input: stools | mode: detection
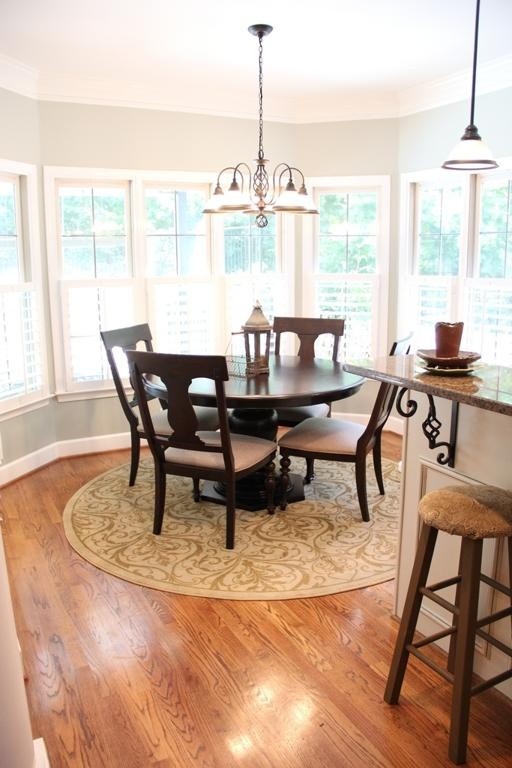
[384,484,512,765]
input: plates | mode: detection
[416,349,482,365]
[418,360,475,377]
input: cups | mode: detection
[434,321,464,358]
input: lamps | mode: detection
[201,23,320,229]
[440,0,500,172]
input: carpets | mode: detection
[60,447,402,601]
